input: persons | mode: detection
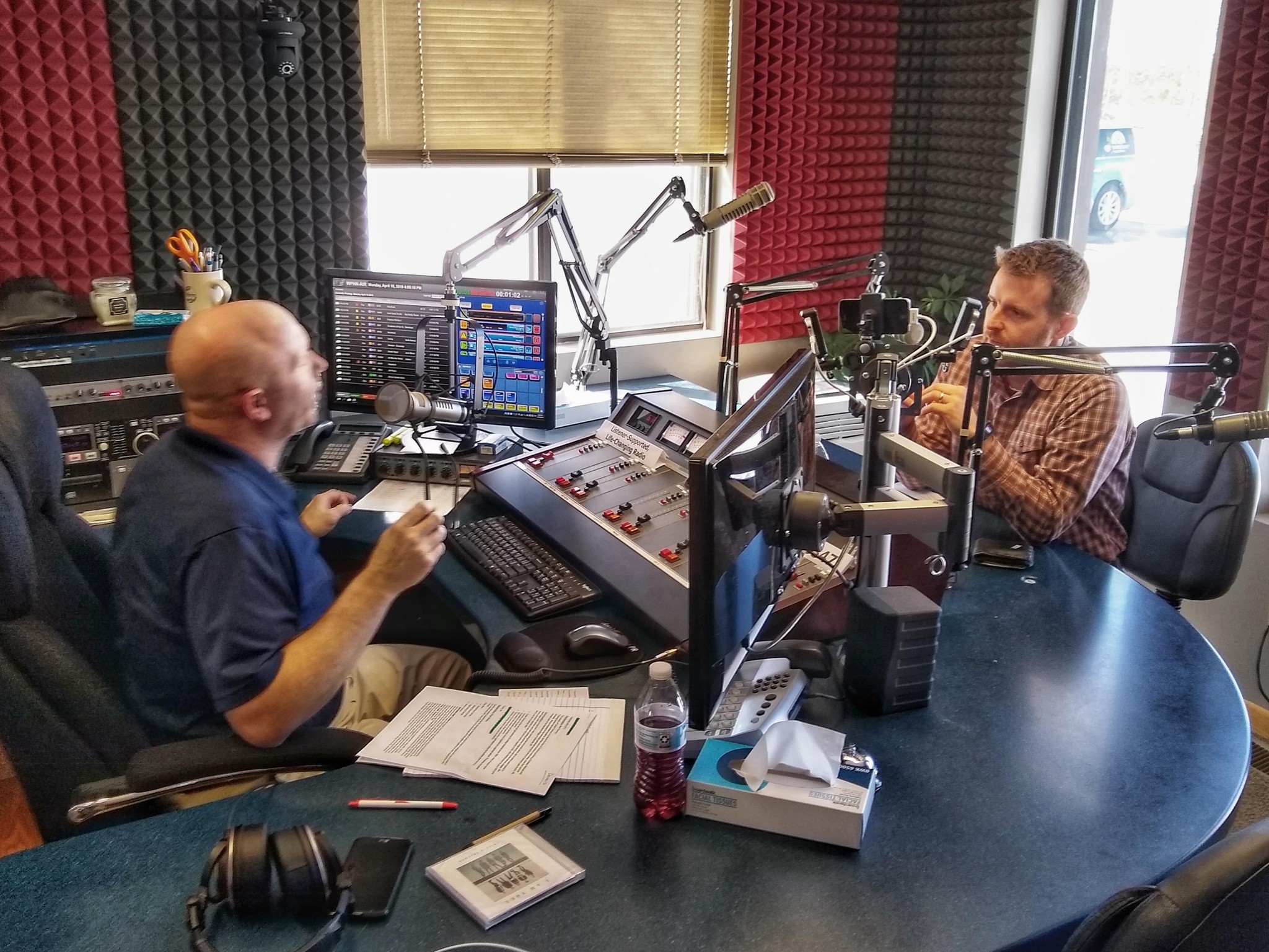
[472,849,535,894]
[895,239,1137,574]
[110,300,471,811]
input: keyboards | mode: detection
[447,512,602,624]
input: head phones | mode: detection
[183,823,355,950]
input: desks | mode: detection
[0,377,1251,952]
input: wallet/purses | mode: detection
[971,538,1035,570]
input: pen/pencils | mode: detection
[463,807,556,852]
[347,799,460,808]
[178,239,224,272]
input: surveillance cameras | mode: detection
[259,20,305,79]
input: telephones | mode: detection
[644,637,833,755]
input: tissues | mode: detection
[685,720,879,852]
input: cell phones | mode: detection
[336,835,411,920]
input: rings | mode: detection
[939,393,945,404]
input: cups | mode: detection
[182,268,232,315]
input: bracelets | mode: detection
[964,423,994,446]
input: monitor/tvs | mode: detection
[687,341,831,732]
[322,268,557,430]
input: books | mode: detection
[425,822,586,930]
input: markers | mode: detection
[382,423,411,446]
[167,227,201,266]
[394,428,413,445]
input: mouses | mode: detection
[562,622,630,659]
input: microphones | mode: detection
[374,381,470,425]
[701,183,775,231]
[1152,410,1269,442]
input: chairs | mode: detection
[1110,415,1261,613]
[1061,821,1269,952]
[0,362,372,850]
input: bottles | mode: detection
[89,276,136,326]
[635,661,689,820]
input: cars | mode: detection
[1086,128,1139,232]
